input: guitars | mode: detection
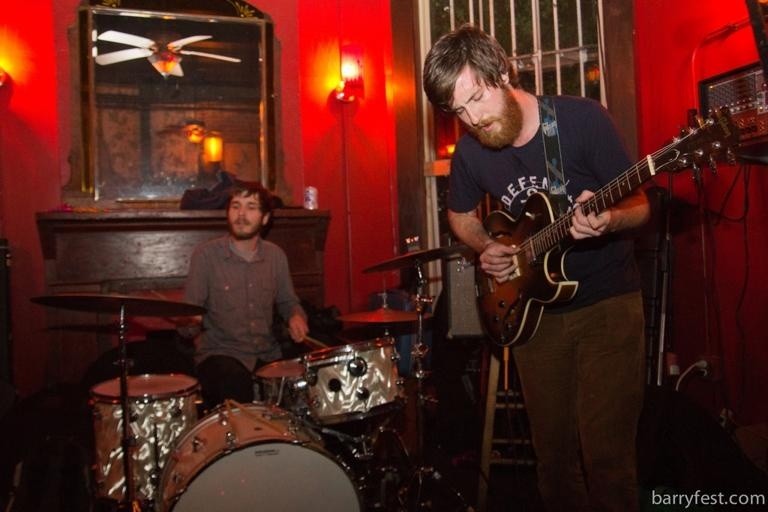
[473,107,741,347]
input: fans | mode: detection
[95,21,242,80]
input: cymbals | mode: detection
[30,291,208,317]
[336,307,432,323]
[362,245,470,272]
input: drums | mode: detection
[152,402,368,512]
[253,358,306,418]
[304,336,407,426]
[90,374,204,502]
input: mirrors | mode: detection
[80,6,272,210]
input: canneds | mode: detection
[303,185,319,210]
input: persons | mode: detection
[173,180,309,413]
[422,21,653,511]
